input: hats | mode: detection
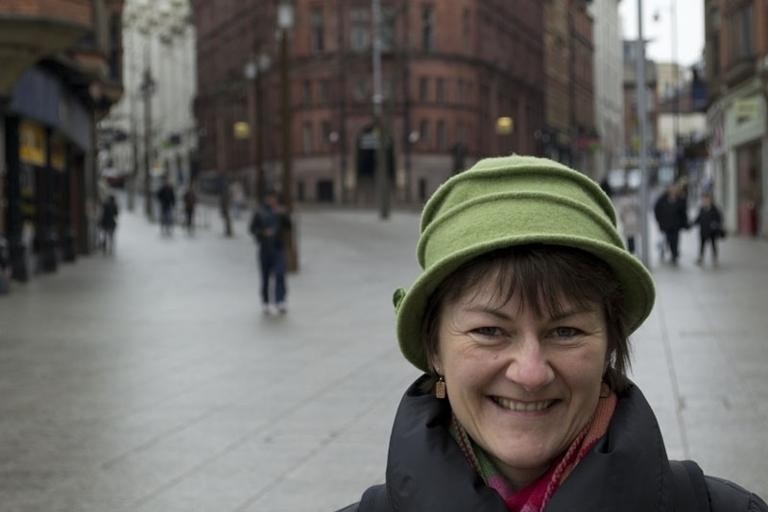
[395,154,655,375]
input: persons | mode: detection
[336,151,767,511]
[615,170,727,269]
[249,188,293,316]
[154,172,245,239]
[99,195,118,253]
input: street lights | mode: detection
[137,67,158,213]
[276,5,296,205]
[242,55,272,199]
